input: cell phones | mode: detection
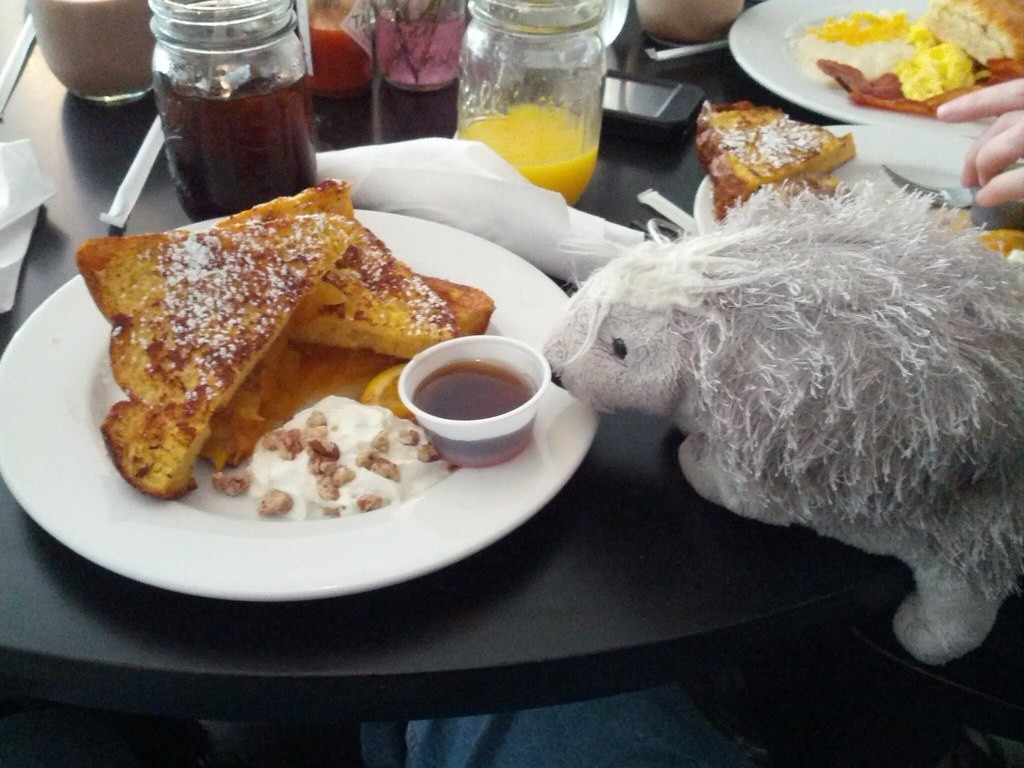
[552,66,706,149]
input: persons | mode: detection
[937,77,1024,206]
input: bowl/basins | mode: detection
[397,330,554,467]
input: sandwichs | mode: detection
[79,178,493,501]
[698,98,859,220]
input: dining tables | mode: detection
[0,1,1022,768]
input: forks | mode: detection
[879,162,981,211]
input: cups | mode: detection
[288,0,373,98]
[145,1,318,223]
[26,0,157,102]
[454,1,605,208]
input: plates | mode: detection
[694,122,1024,278]
[724,0,1024,127]
[2,207,602,602]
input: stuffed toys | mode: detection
[543,177,1024,665]
